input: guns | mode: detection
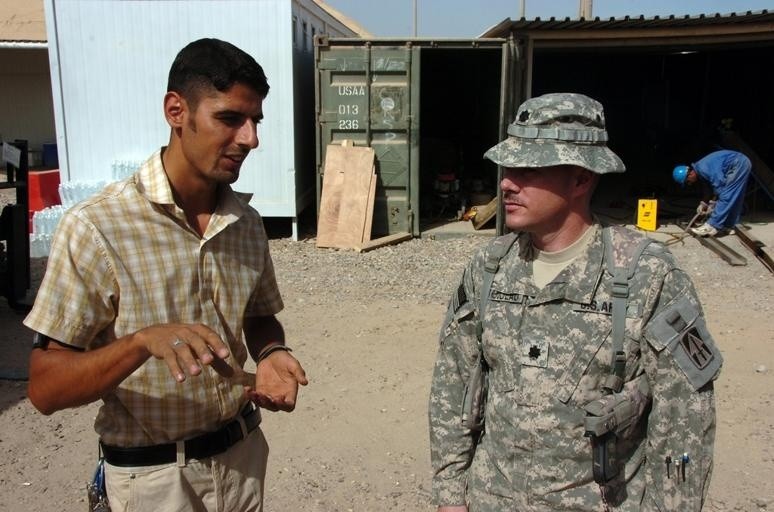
[584,393,626,483]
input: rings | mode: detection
[174,338,184,346]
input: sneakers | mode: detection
[690,223,722,237]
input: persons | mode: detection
[673,148,752,237]
[22,36,308,511]
[427,90,723,511]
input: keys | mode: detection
[83,479,111,511]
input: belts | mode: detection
[99,397,262,467]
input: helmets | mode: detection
[672,165,690,189]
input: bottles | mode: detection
[29,159,148,259]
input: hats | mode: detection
[481,91,628,174]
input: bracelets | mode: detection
[256,344,293,364]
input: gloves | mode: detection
[696,199,717,216]
